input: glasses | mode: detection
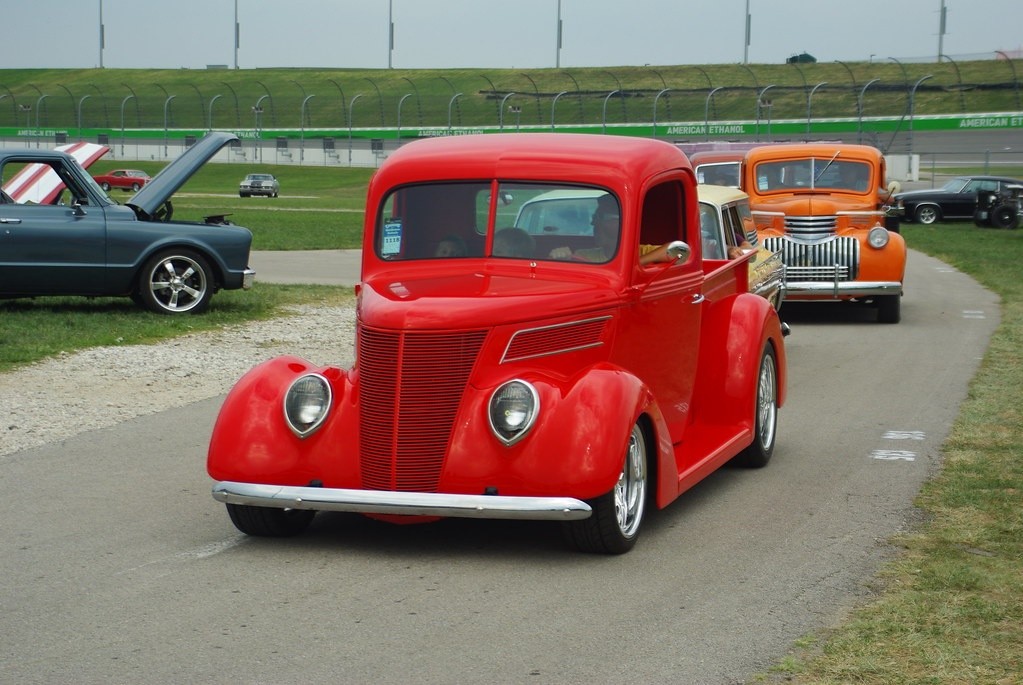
[591,211,619,226]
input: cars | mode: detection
[728,141,907,325]
[690,148,795,197]
[92,169,152,193]
[785,54,816,64]
[889,174,1023,226]
[205,133,791,559]
[0,132,256,317]
[513,179,789,314]
[239,173,280,198]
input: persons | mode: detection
[703,237,744,260]
[996,184,1012,198]
[703,209,756,264]
[435,234,466,257]
[550,193,676,265]
[714,175,727,185]
[833,162,894,205]
[493,228,536,258]
[121,171,129,178]
